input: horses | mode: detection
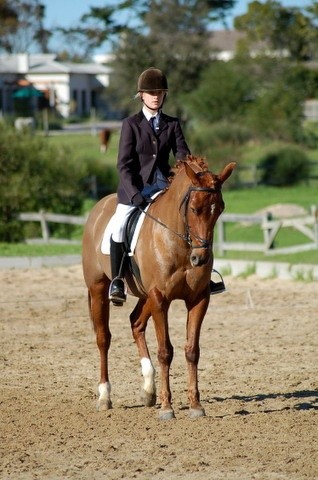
[97,129,110,152]
[82,154,236,419]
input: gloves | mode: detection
[132,193,146,206]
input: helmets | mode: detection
[137,67,169,91]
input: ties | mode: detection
[149,117,156,136]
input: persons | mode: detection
[109,67,225,307]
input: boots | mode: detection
[110,233,126,306]
[210,279,225,294]
